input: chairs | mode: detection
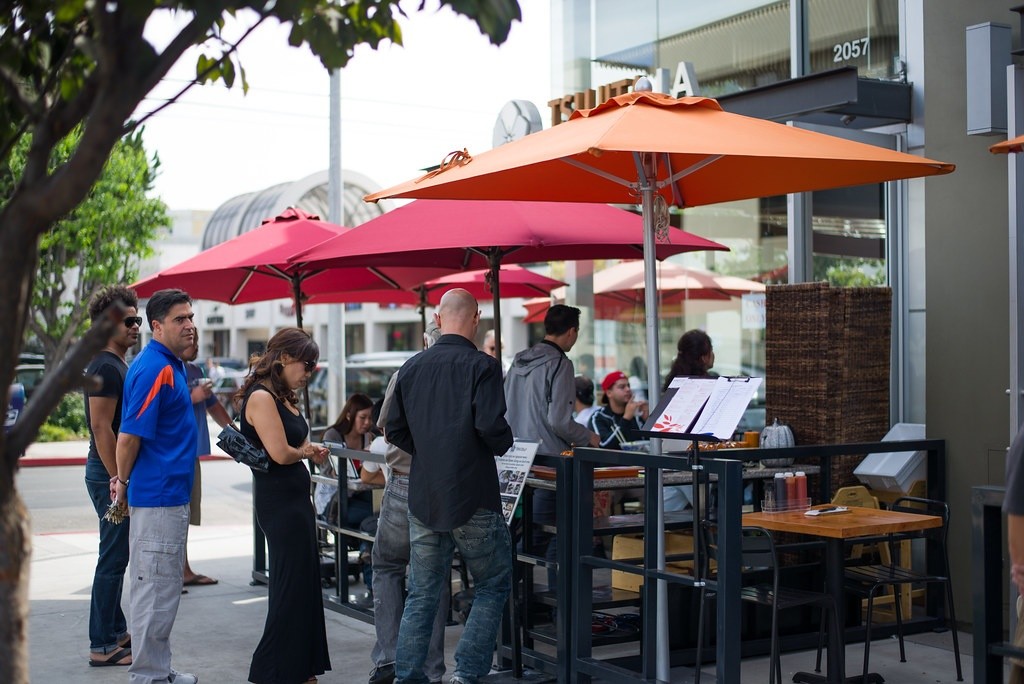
[696,490,963,684]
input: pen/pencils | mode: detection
[818,507,837,512]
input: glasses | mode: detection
[303,361,316,374]
[112,316,143,328]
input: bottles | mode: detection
[775,471,807,512]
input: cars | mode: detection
[191,357,246,421]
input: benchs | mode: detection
[318,502,721,650]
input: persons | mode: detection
[109,289,241,684]
[84,284,143,668]
[313,325,506,684]
[233,326,331,684]
[503,305,752,513]
[383,287,514,684]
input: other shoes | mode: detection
[156,670,198,684]
[369,665,395,684]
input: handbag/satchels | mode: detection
[216,424,273,474]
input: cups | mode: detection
[199,378,211,399]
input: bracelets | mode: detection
[118,478,129,485]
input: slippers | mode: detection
[89,648,135,666]
[117,637,133,648]
[182,573,219,584]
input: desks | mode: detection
[311,474,385,596]
[740,504,942,684]
[522,465,821,649]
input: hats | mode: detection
[601,372,627,404]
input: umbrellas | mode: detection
[126,198,770,453]
[362,78,954,682]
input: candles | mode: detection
[744,429,759,447]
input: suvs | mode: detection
[299,351,419,423]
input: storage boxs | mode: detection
[853,423,927,493]
